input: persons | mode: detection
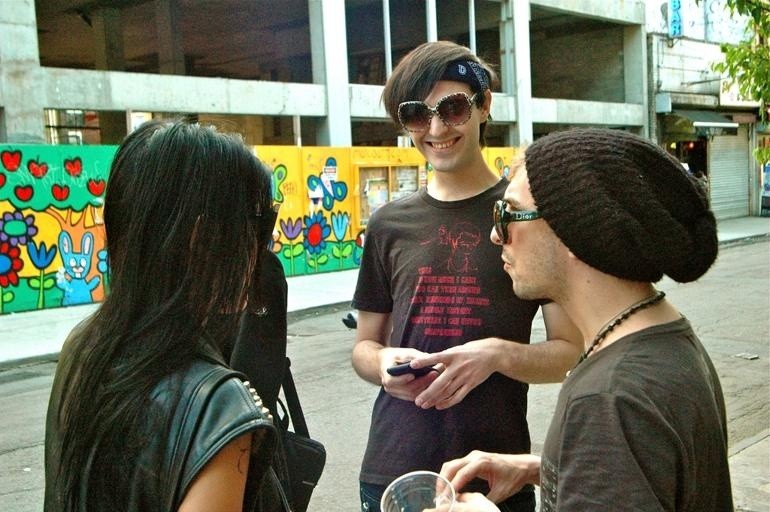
[44,117,277,512]
[423,127,732,511]
[348,41,586,511]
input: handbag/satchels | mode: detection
[270,429,326,511]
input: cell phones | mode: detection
[387,362,429,377]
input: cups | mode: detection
[379,469,456,512]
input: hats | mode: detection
[525,128,718,282]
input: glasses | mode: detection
[493,199,542,244]
[397,92,478,133]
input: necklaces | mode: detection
[572,289,665,368]
